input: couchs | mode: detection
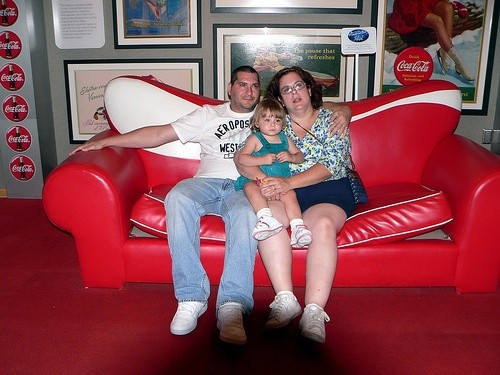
[42,75,500,294]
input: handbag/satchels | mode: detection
[346,170,368,204]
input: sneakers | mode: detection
[300,303,330,344]
[170,301,208,335]
[217,302,247,343]
[266,292,302,328]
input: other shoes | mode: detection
[252,215,283,241]
[290,225,312,248]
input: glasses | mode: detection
[279,81,306,96]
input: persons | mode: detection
[234,66,356,342]
[69,65,353,345]
[238,98,312,248]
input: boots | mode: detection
[436,47,450,72]
[448,46,475,81]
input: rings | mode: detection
[342,125,346,128]
[272,185,276,190]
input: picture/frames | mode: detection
[213,23,359,102]
[63,58,203,144]
[112,0,202,49]
[367,0,500,116]
[210,0,363,15]
